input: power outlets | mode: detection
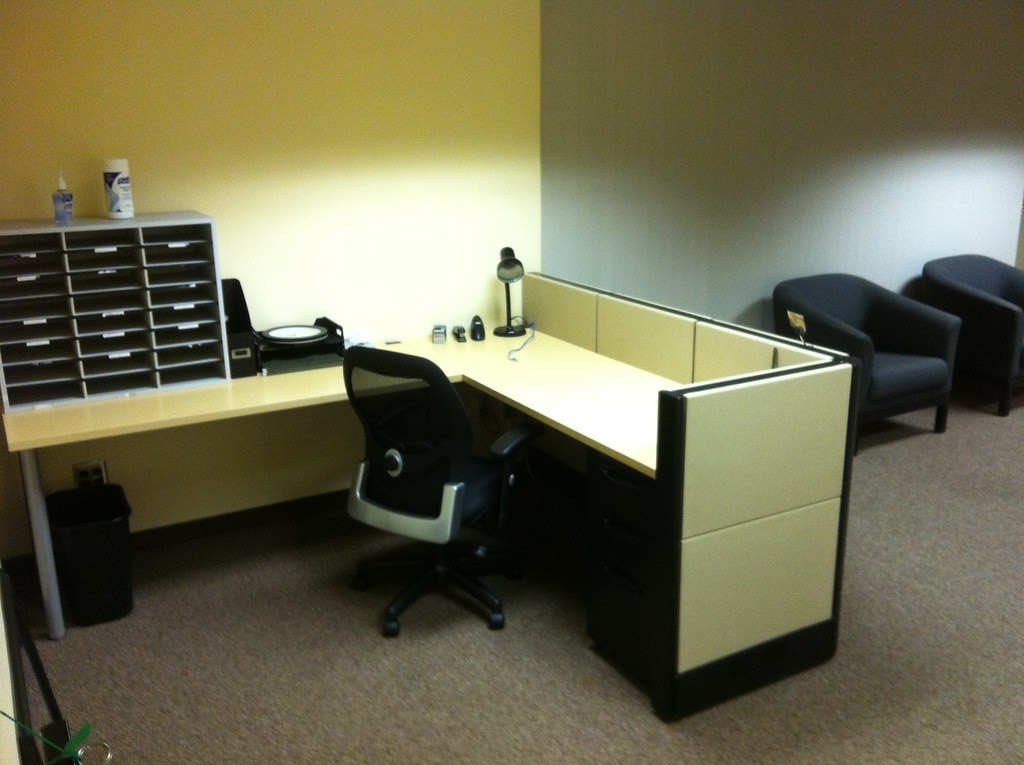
[72,459,107,488]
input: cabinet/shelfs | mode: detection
[1,209,232,412]
[586,447,657,695]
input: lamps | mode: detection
[494,246,526,337]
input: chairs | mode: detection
[923,254,1024,416]
[773,272,962,455]
[343,345,536,636]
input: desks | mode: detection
[0,270,861,723]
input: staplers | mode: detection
[452,325,467,343]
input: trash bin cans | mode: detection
[44,483,134,627]
